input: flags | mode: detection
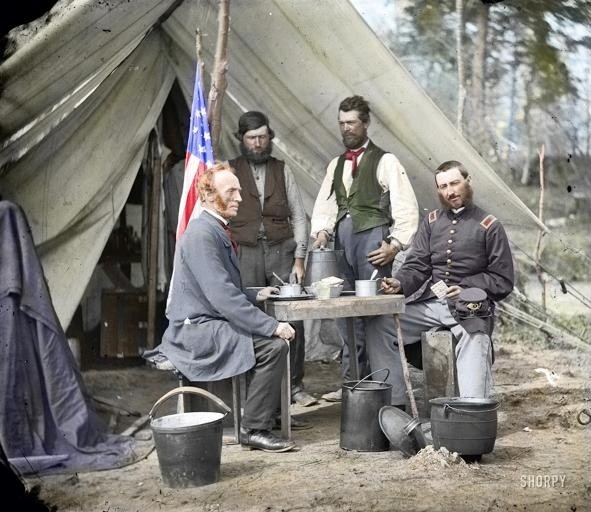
[177,66,215,246]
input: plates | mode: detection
[267,294,315,300]
[341,291,356,295]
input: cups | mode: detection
[356,278,386,297]
[318,282,331,300]
[274,284,302,296]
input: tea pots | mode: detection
[305,246,354,295]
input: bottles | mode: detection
[111,225,144,251]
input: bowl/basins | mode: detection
[303,286,344,298]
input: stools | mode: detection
[173,370,254,448]
[417,326,464,404]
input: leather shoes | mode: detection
[289,383,317,407]
[273,414,313,431]
[321,391,342,402]
[240,428,296,453]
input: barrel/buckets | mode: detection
[302,247,340,287]
[339,379,392,452]
[149,411,226,489]
[429,395,495,454]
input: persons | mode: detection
[158,163,295,451]
[365,161,514,412]
[225,111,317,406]
[310,95,419,411]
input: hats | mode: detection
[455,287,493,320]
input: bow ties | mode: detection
[342,152,357,180]
[226,226,239,256]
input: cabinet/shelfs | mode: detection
[97,288,168,365]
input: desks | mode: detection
[256,287,427,443]
